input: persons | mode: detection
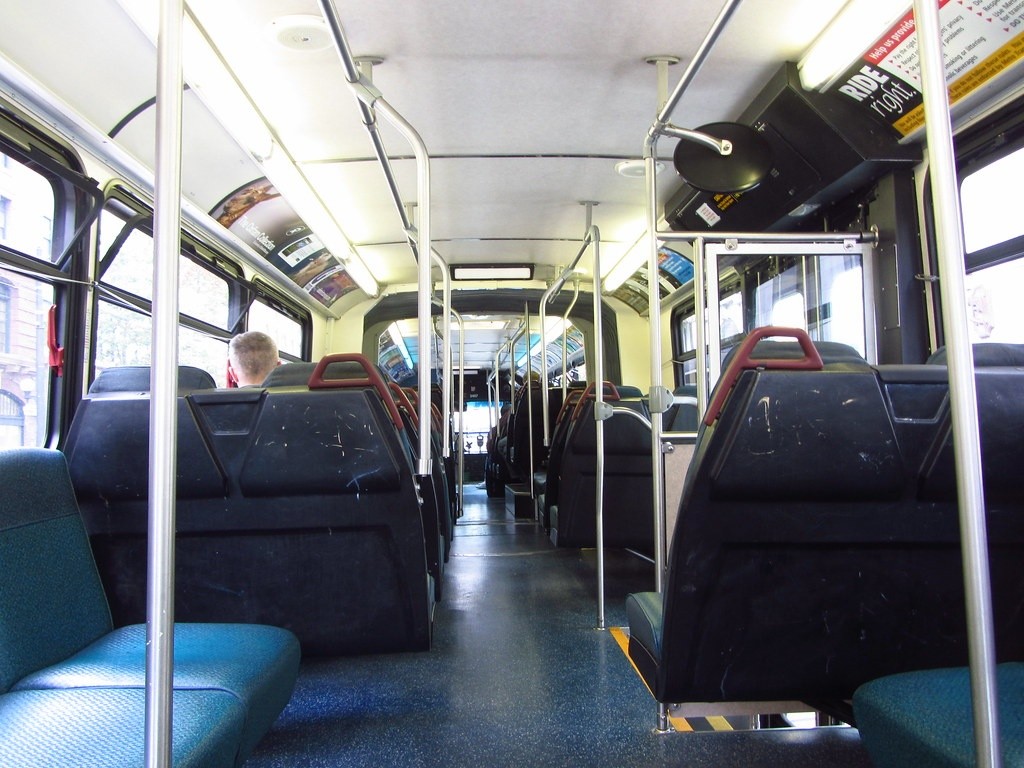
[228,330,282,390]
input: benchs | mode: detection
[626,325,1022,699]
[65,353,458,653]
[852,660,1024,768]
[0,449,300,768]
[485,381,698,546]
